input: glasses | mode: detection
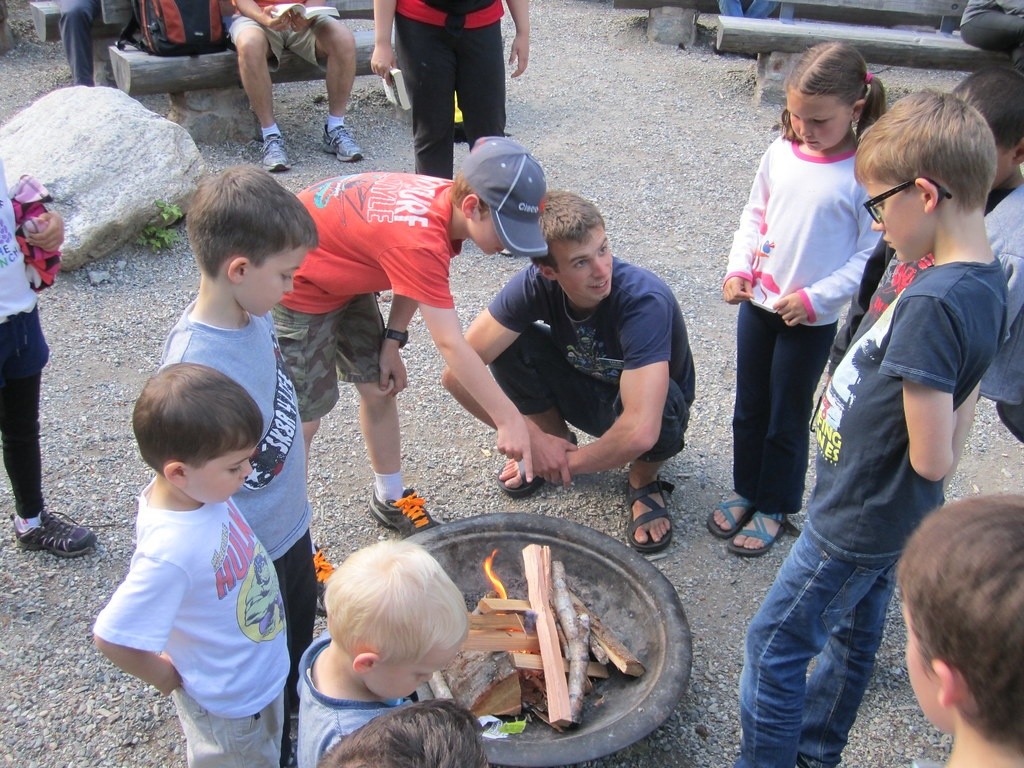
[862,176,953,224]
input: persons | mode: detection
[898,496,1024,768]
[159,165,318,768]
[58,0,95,86]
[0,157,96,558]
[92,364,290,768]
[961,1,1024,73]
[371,0,529,256]
[319,700,489,768]
[297,541,469,768]
[224,0,364,171]
[442,189,695,552]
[707,41,885,557]
[273,140,550,619]
[734,68,1024,768]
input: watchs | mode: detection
[384,328,408,348]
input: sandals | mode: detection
[495,431,578,497]
[626,472,677,551]
[726,509,787,555]
[706,491,755,539]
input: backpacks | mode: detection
[117,0,232,58]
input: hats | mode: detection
[462,136,549,257]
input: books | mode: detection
[382,67,410,110]
[270,3,340,20]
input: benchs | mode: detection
[614,0,1011,108]
[30,0,402,145]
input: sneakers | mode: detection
[259,129,291,172]
[367,486,442,539]
[321,123,363,162]
[10,504,98,558]
[311,544,336,616]
